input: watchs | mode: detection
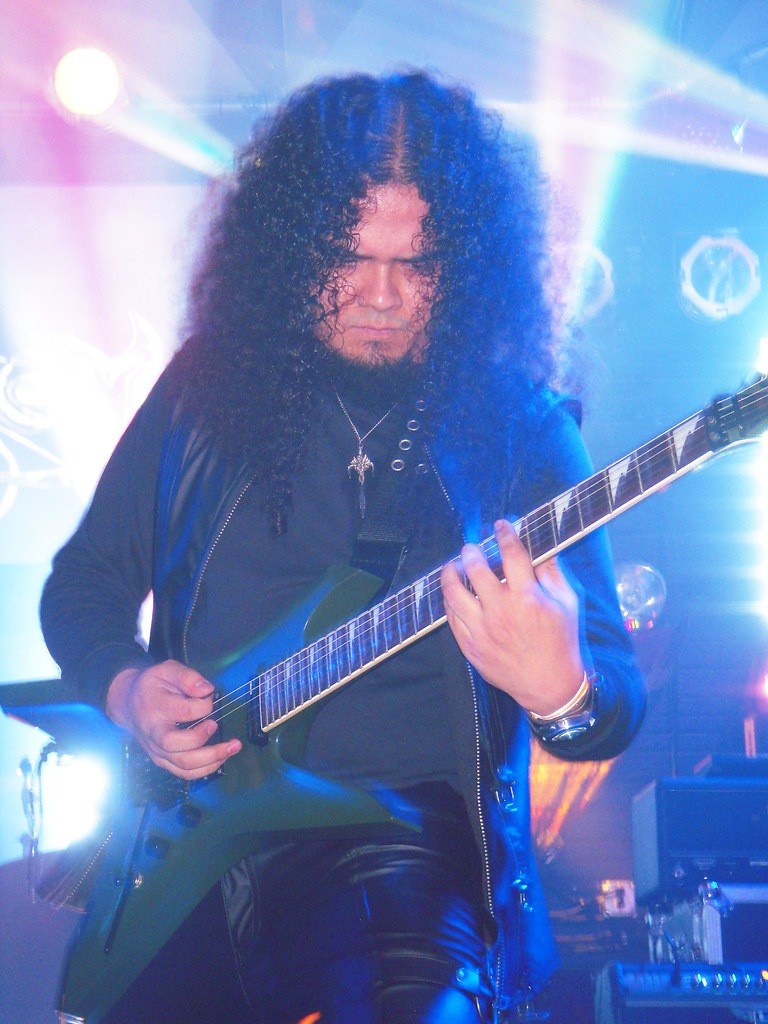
[521,673,599,744]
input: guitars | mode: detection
[2,376,766,1023]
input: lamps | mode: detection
[55,43,122,118]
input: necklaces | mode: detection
[323,371,407,487]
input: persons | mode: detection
[40,65,648,1024]
